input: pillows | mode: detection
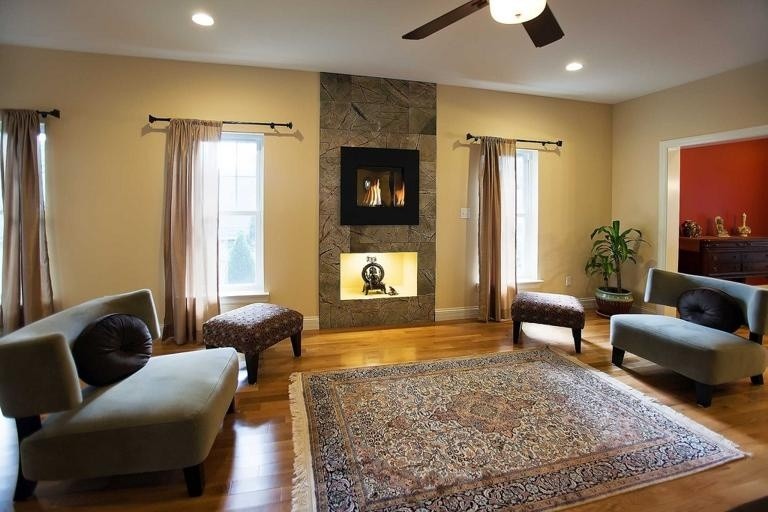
[677,287,744,334]
[72,313,153,388]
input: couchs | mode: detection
[609,268,768,409]
[0,288,239,502]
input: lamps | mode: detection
[487,0,547,25]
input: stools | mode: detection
[511,291,585,354]
[202,302,304,384]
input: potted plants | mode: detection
[584,220,651,319]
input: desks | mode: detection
[679,235,768,279]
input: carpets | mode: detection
[287,342,751,512]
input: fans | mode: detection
[401,0,565,48]
